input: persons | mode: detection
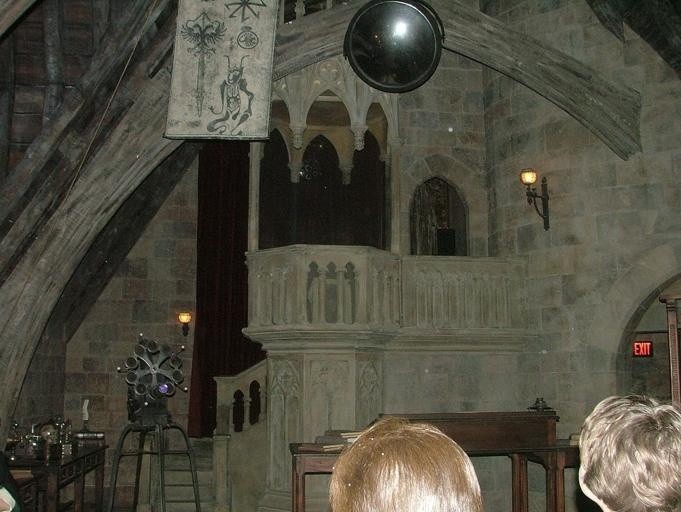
[0,450,24,512]
[574,391,681,512]
[328,415,485,512]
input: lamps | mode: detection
[518,169,550,231]
[178,311,191,337]
